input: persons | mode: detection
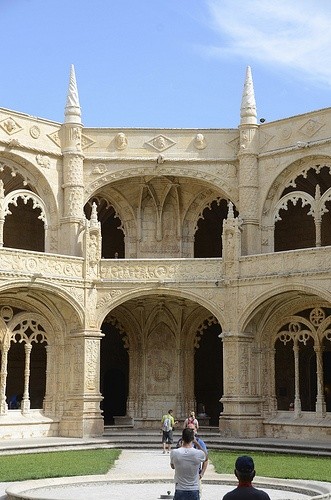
[183,411,198,430]
[170,428,208,500]
[222,455,270,500]
[160,409,178,454]
[176,422,208,499]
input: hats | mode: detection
[235,455,256,475]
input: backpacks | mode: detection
[186,419,196,434]
[162,415,173,432]
